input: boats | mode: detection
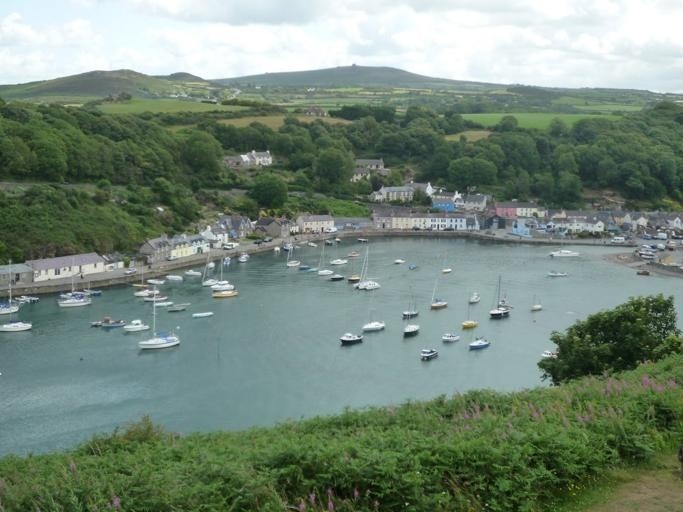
[418,345,438,361]
[272,246,280,252]
[191,312,213,319]
[408,263,418,270]
[237,250,249,262]
[146,277,165,285]
[541,351,558,359]
[546,270,567,277]
[440,267,451,274]
[468,335,490,349]
[0,322,33,332]
[529,304,542,311]
[87,314,150,331]
[165,274,183,281]
[15,295,39,304]
[182,269,201,277]
[143,295,185,312]
[461,319,479,328]
[360,320,384,331]
[439,333,459,342]
[338,332,363,342]
[469,289,480,304]
[393,257,407,264]
[636,270,650,275]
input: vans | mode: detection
[166,255,176,260]
[610,237,624,244]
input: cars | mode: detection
[410,226,454,232]
[263,237,272,242]
[638,232,682,260]
[253,239,262,244]
[223,243,233,250]
[124,267,136,275]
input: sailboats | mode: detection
[137,288,179,349]
[55,272,101,307]
[201,252,238,297]
[131,259,160,297]
[487,275,513,317]
[429,278,447,309]
[400,285,419,335]
[0,259,22,315]
[280,214,380,290]
[549,220,584,258]
